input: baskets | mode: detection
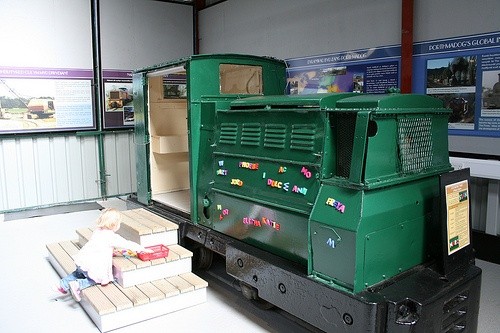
[137,243,170,261]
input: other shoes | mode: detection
[69,280,81,302]
[52,282,68,296]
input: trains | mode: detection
[124,52,482,333]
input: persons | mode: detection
[57,208,154,302]
